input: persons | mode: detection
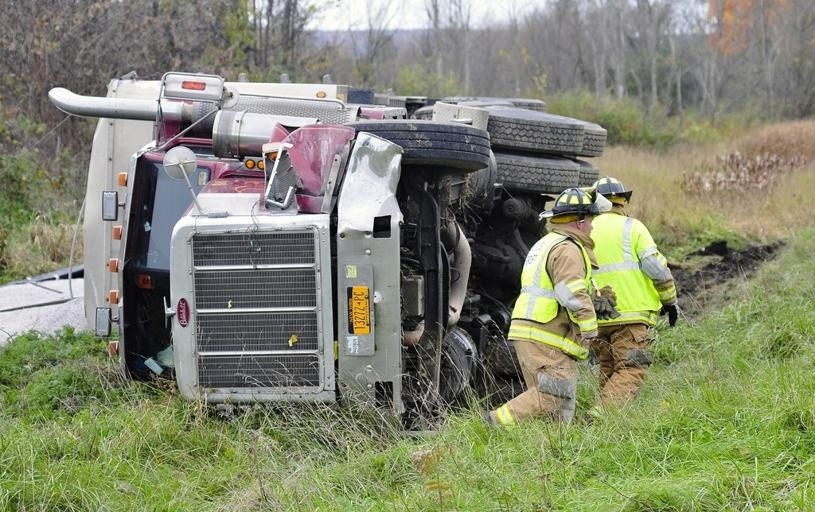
[460,184,620,426]
[583,174,680,424]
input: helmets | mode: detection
[538,177,632,224]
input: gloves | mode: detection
[660,303,680,326]
[592,286,620,320]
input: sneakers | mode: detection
[487,411,512,441]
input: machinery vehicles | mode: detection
[48,67,610,437]
